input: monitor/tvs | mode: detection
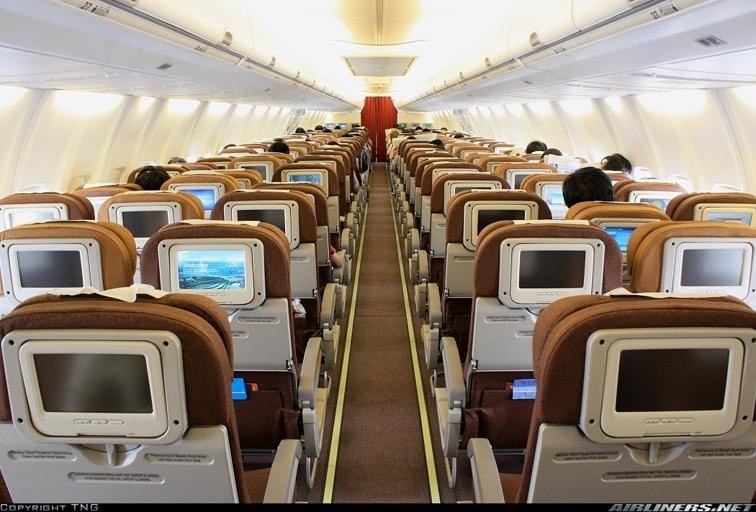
[338,123,347,128]
[325,122,336,129]
[174,185,220,220]
[17,341,169,437]
[86,196,113,222]
[285,172,324,188]
[169,243,255,305]
[600,337,745,437]
[510,243,595,304]
[495,146,510,154]
[636,193,676,214]
[471,204,531,245]
[511,172,546,189]
[450,183,495,198]
[238,164,270,182]
[535,180,570,219]
[7,243,92,302]
[600,221,648,252]
[701,207,755,227]
[115,205,174,247]
[232,203,291,245]
[2,207,61,231]
[672,242,753,301]
[216,164,226,170]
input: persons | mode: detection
[222,122,369,268]
[597,154,631,173]
[168,157,187,164]
[383,124,465,172]
[538,148,563,163]
[562,167,614,210]
[525,140,547,155]
[134,166,170,190]
